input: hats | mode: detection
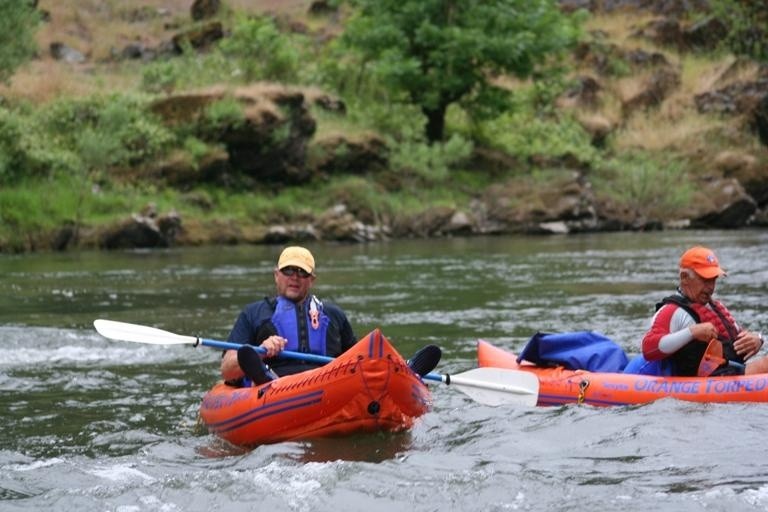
[278,245,316,278]
[679,247,727,278]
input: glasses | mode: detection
[280,269,307,276]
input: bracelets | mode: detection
[757,333,764,345]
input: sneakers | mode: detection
[237,346,270,382]
[408,345,442,374]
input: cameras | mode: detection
[722,339,744,363]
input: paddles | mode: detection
[93,319,539,406]
[698,338,746,377]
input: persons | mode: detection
[220,246,444,391]
[641,246,766,377]
